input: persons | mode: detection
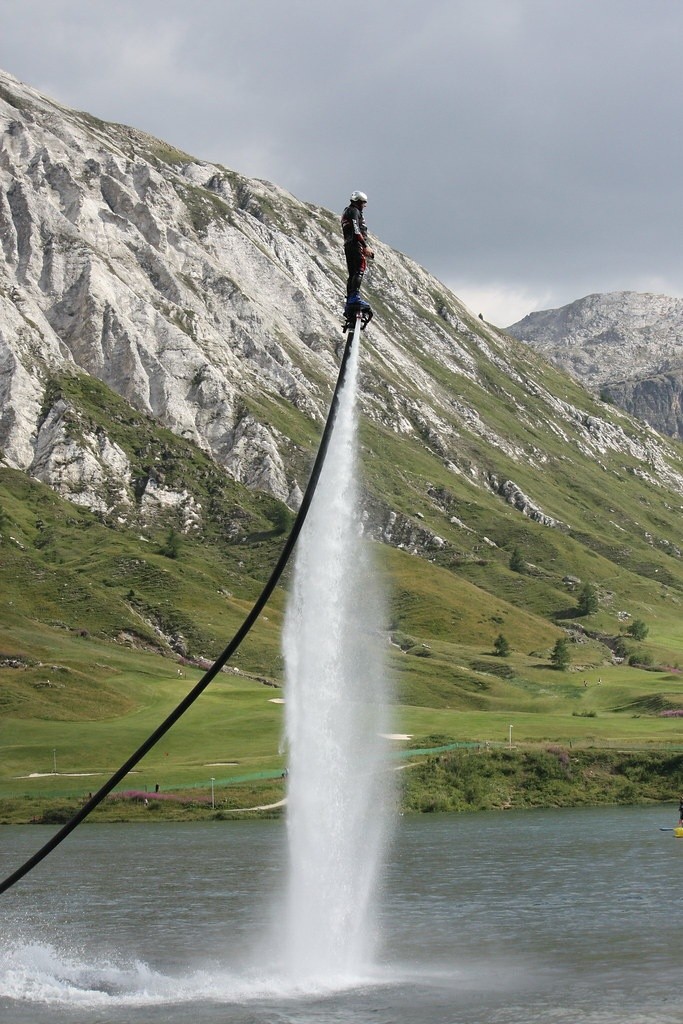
[678,795,683,828]
[584,681,587,687]
[144,797,148,806]
[341,191,374,308]
[284,768,288,777]
[155,783,159,792]
[598,677,601,685]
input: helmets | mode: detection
[350,190,367,203]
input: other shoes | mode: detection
[347,290,367,305]
[345,304,349,312]
